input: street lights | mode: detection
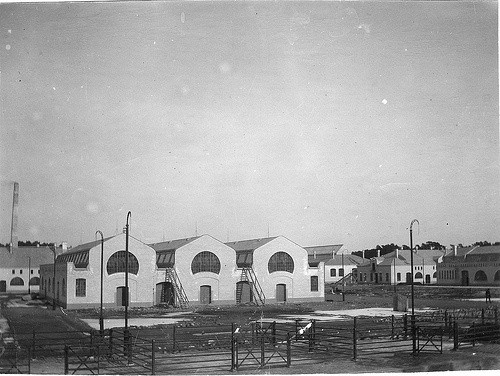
[122,211,133,354]
[341,249,349,301]
[48,243,56,310]
[26,254,31,294]
[409,219,422,356]
[92,230,103,336]
